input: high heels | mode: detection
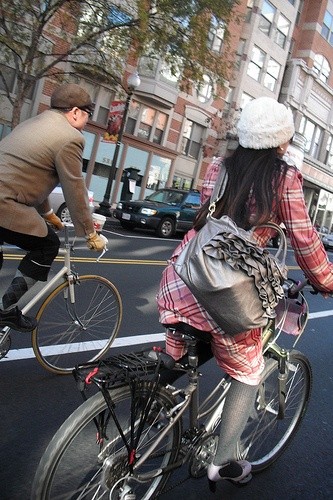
[207,459,252,493]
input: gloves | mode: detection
[84,229,105,252]
[39,208,65,232]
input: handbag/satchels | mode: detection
[172,221,290,334]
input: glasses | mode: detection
[79,107,93,118]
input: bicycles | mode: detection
[0,224,123,376]
[29,279,333,500]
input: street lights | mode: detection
[95,69,141,217]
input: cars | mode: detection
[47,185,96,225]
[272,222,287,248]
[322,231,333,251]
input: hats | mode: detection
[51,84,96,109]
[236,96,295,150]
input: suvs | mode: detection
[113,188,202,239]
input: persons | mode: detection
[0,84,109,332]
[157,98,333,494]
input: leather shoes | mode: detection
[0,305,38,333]
